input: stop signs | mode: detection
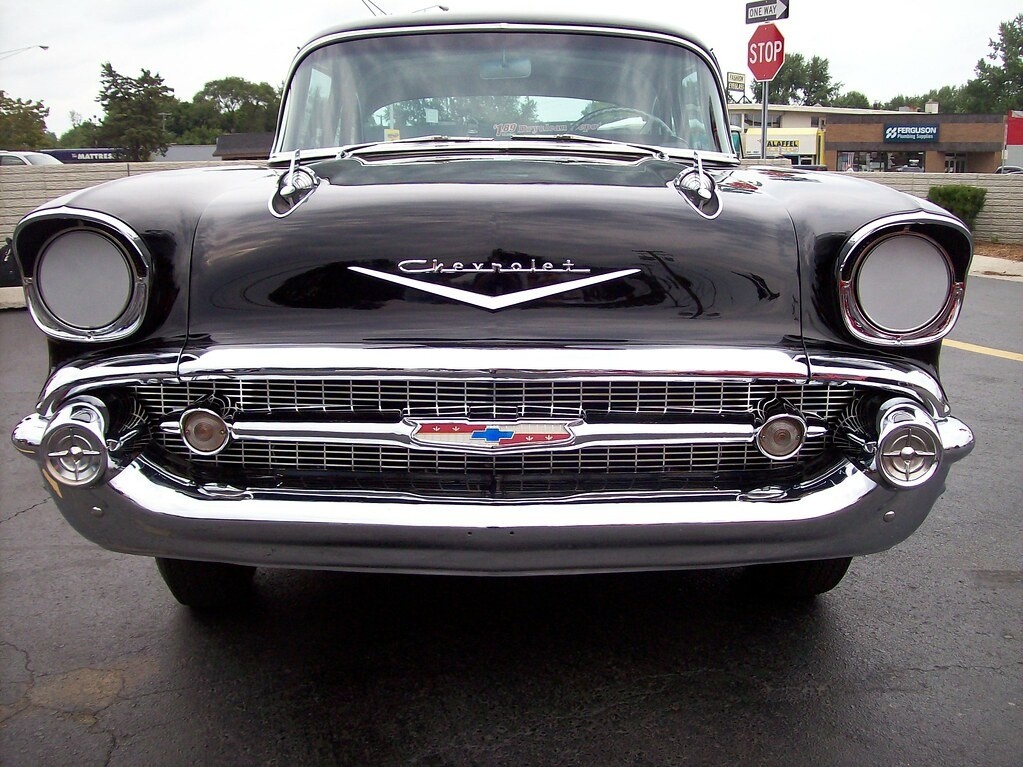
[748,22,785,82]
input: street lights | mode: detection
[158,112,173,131]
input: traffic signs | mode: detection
[745,0,791,24]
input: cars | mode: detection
[0,12,976,617]
[0,150,64,166]
